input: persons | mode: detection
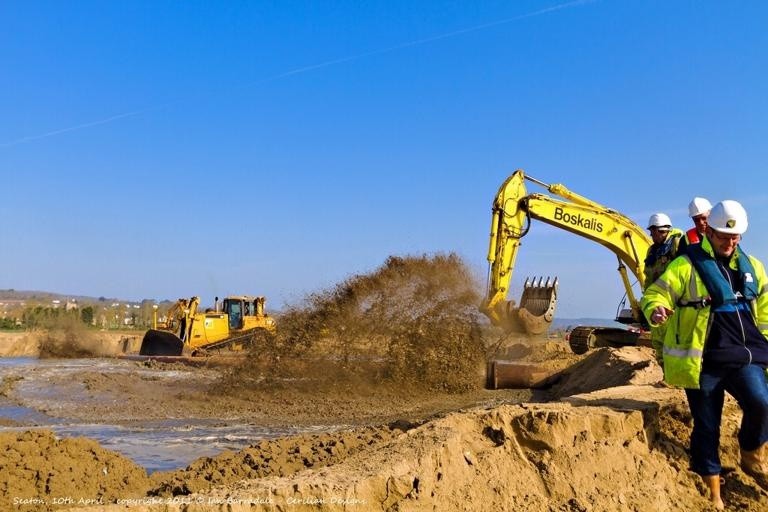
[641,200,768,509]
[676,195,712,255]
[643,210,681,361]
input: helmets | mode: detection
[689,197,712,217]
[707,201,748,235]
[647,214,672,229]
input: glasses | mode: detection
[716,231,743,244]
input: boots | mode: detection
[701,474,724,510]
[740,444,768,490]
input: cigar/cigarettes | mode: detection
[656,313,663,318]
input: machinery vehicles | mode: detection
[477,169,665,355]
[139,296,275,357]
